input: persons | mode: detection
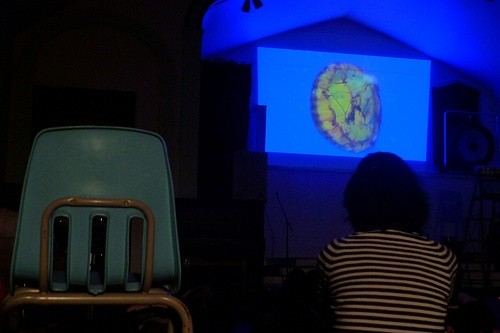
[313,151,458,332]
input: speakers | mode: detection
[433,80,479,171]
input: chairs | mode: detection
[0,125,193,333]
